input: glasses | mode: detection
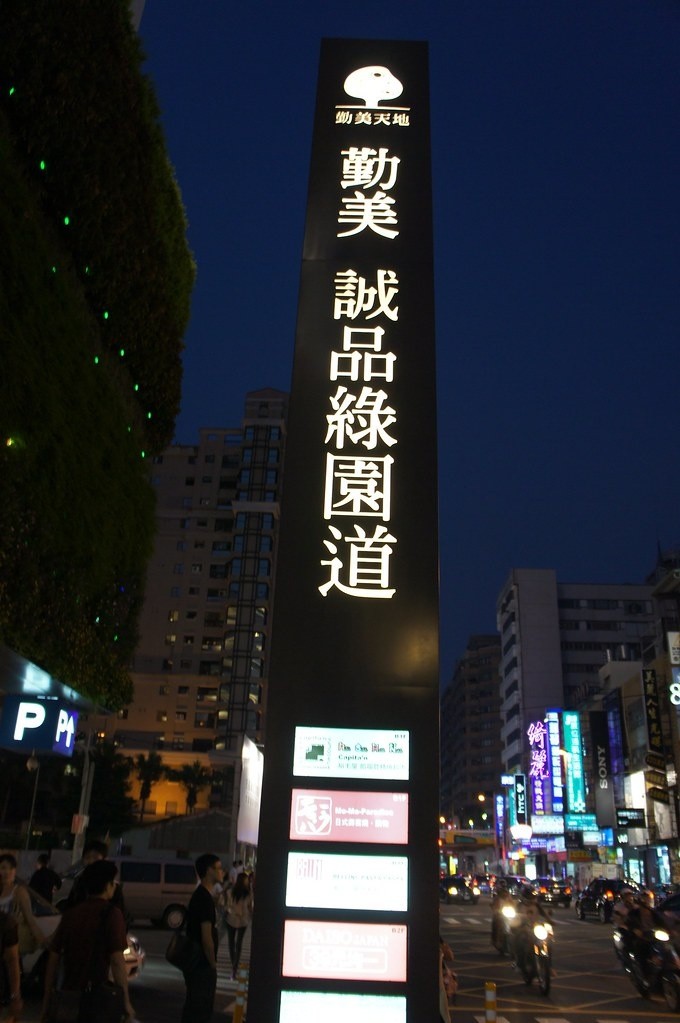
[113,880,120,888]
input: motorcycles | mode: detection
[490,904,521,964]
[511,907,556,997]
[609,924,680,1014]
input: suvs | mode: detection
[574,874,655,924]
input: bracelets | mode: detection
[11,992,20,999]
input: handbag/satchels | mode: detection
[166,933,206,975]
[78,978,124,1022]
[215,911,226,930]
[12,887,39,955]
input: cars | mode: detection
[653,891,680,925]
[474,872,536,905]
[440,876,480,906]
[532,875,572,909]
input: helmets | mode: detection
[638,889,654,905]
[620,889,633,898]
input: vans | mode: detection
[47,852,205,933]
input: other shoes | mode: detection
[551,969,559,977]
[228,975,236,981]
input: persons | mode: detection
[0,853,45,1006]
[225,873,253,981]
[490,880,559,978]
[64,840,130,935]
[181,854,224,1023]
[0,875,23,1023]
[612,888,678,984]
[228,861,252,889]
[39,861,136,1023]
[27,854,63,905]
